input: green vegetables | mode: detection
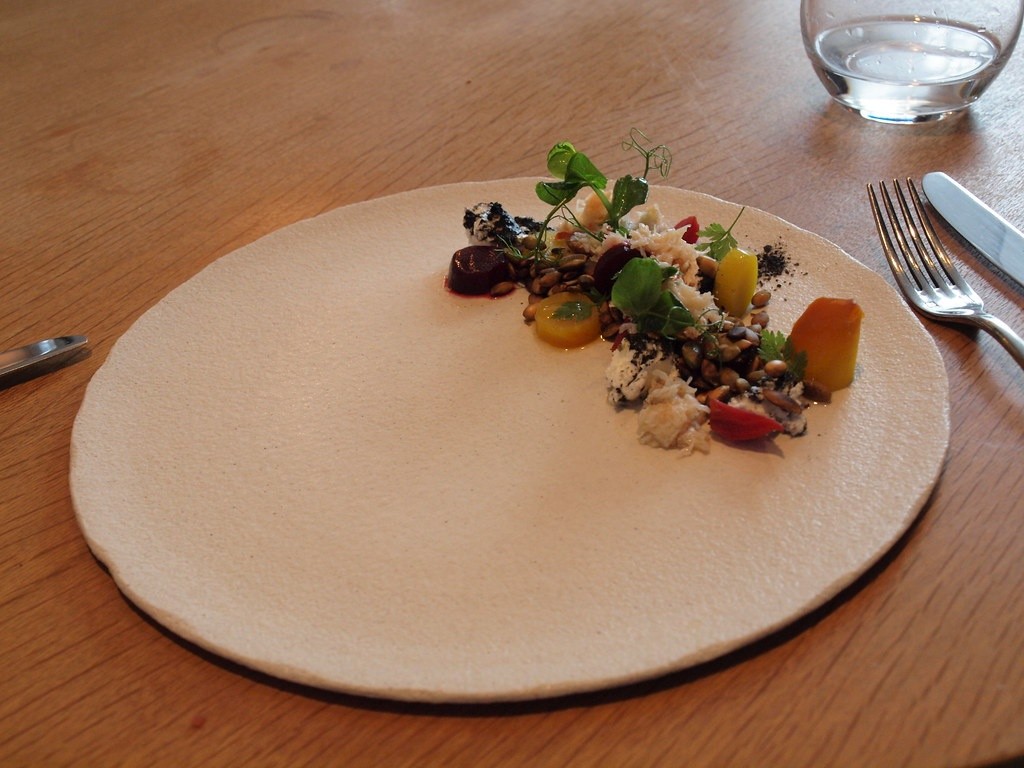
[491,126,808,388]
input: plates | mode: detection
[67,177,951,705]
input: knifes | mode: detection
[0,334,88,382]
[919,170,1024,290]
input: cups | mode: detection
[799,2,1023,129]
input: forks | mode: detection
[868,176,1024,385]
